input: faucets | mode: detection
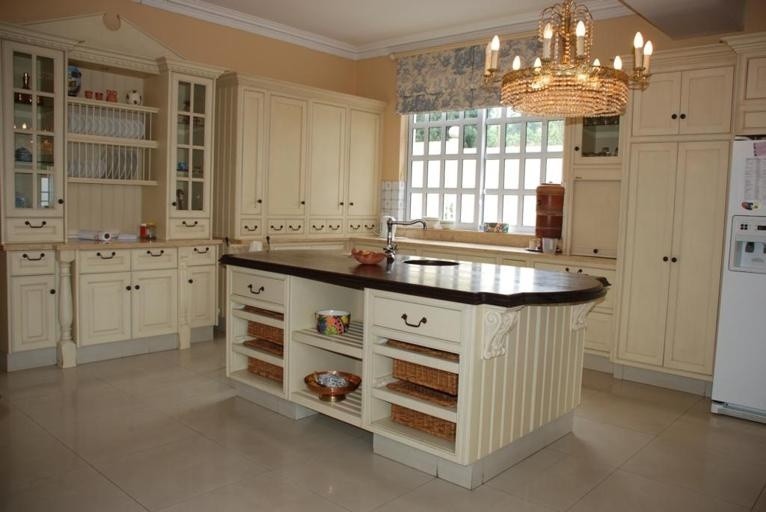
[382,216,428,264]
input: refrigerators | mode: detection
[712,140,766,426]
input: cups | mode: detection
[528,237,561,255]
[84,90,119,103]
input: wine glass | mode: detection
[303,370,360,402]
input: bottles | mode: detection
[137,223,163,241]
[23,72,29,90]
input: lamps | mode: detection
[481,0,653,120]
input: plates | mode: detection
[68,110,159,180]
[15,192,28,207]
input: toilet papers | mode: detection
[78,229,111,241]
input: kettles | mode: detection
[379,216,397,238]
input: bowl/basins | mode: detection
[313,310,351,337]
[423,218,454,231]
[351,247,386,265]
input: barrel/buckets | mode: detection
[535,181,565,239]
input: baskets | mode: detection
[247,320,283,346]
[386,359,457,396]
[246,356,283,384]
[389,404,456,442]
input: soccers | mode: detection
[125,89,143,105]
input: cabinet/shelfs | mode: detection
[226,265,287,399]
[75,250,178,344]
[180,245,219,327]
[366,287,462,459]
[308,85,386,235]
[270,79,306,237]
[214,71,265,241]
[66,53,166,243]
[167,72,214,241]
[1,41,65,245]
[532,256,617,361]
[288,274,364,426]
[6,247,56,353]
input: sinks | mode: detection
[403,259,460,266]
